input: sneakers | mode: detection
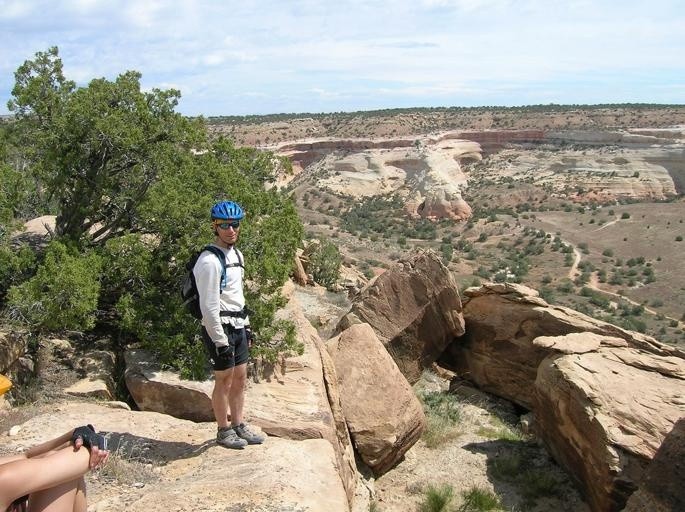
[215,426,248,447]
[231,423,265,444]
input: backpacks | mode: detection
[180,245,242,319]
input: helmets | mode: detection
[0,375,13,396]
[211,201,243,220]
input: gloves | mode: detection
[72,423,110,452]
[216,346,235,366]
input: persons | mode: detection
[192,201,267,449]
[0,373,109,512]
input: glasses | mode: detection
[215,221,240,229]
